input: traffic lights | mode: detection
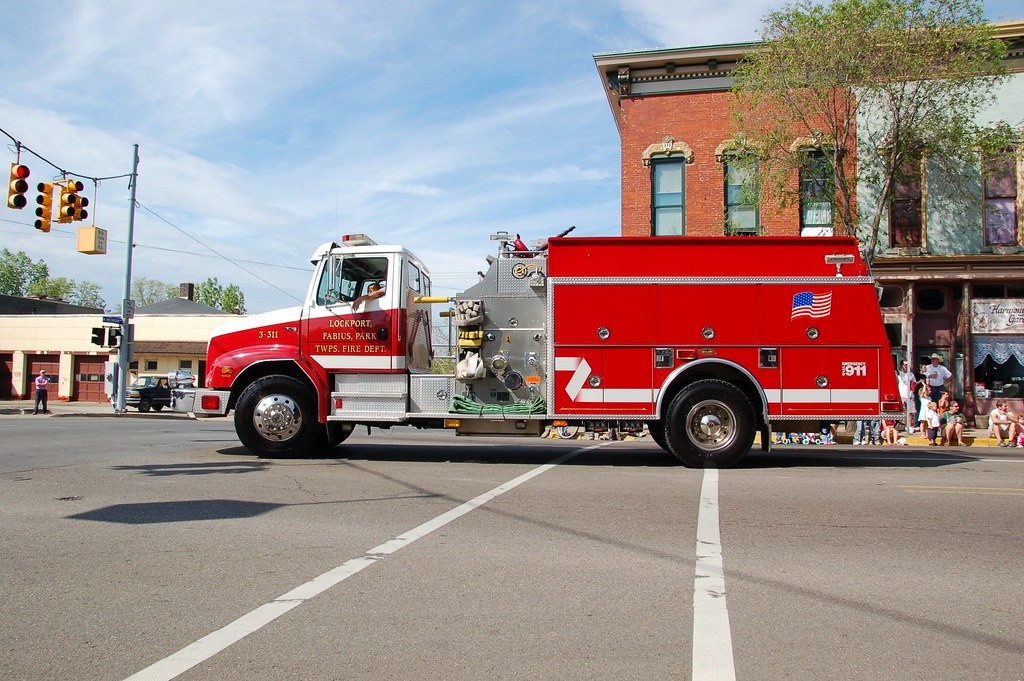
[35,182,52,232]
[74,193,88,221]
[58,190,76,222]
[108,328,118,346]
[7,162,31,210]
[91,328,105,345]
[67,179,84,192]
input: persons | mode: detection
[352,265,387,311]
[557,426,647,440]
[31,370,49,415]
[853,353,968,447]
[776,423,838,445]
[988,399,1024,448]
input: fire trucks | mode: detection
[192,225,910,468]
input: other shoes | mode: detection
[959,442,968,446]
[944,442,950,446]
[1009,441,1015,447]
[44,413,47,415]
[929,442,937,446]
[32,411,36,414]
[999,441,1006,447]
[852,440,897,445]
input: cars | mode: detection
[109,376,195,413]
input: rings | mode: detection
[355,304,358,306]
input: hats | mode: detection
[40,369,44,373]
[929,352,943,362]
